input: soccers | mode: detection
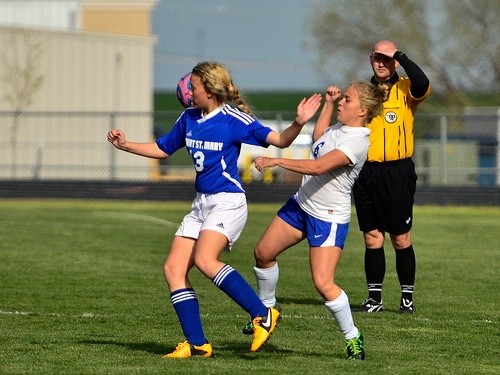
[177,72,194,108]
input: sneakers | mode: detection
[344,329,364,360]
[354,298,385,313]
[243,302,282,334]
[162,339,212,358]
[399,298,416,314]
[250,308,280,352]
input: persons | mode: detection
[107,62,322,359]
[243,81,386,360]
[352,40,431,314]
